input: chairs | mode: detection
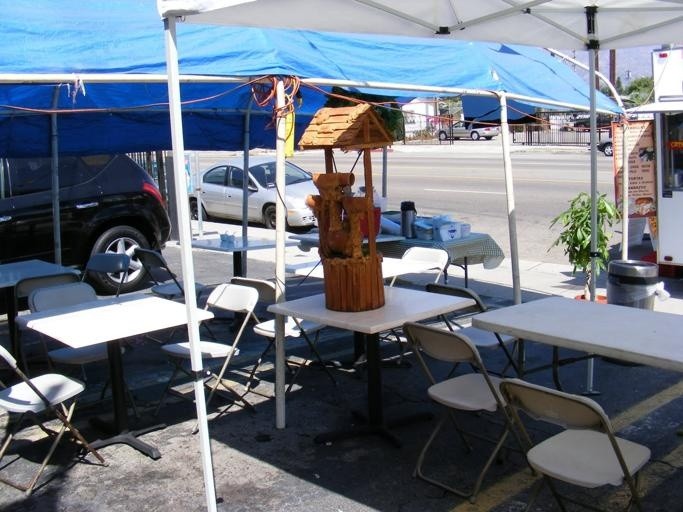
[81,253,131,297]
[402,320,534,500]
[228,275,351,410]
[133,246,217,343]
[500,375,651,510]
[0,343,110,495]
[13,271,79,314]
[425,282,520,379]
[373,243,455,371]
[152,283,260,434]
[27,281,140,420]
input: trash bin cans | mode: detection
[602,260,659,366]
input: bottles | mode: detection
[400,201,415,238]
[431,214,448,243]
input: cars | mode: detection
[597,138,613,156]
[129,155,168,201]
[434,120,499,141]
[186,155,321,231]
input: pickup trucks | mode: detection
[563,113,611,132]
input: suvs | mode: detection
[0,153,172,296]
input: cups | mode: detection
[459,223,471,239]
[219,234,243,245]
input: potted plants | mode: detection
[544,189,625,304]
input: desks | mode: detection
[471,297,681,436]
[178,234,302,333]
[299,210,506,286]
[0,258,81,385]
[290,233,405,247]
[266,283,477,448]
[13,290,215,459]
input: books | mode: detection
[414,219,432,241]
